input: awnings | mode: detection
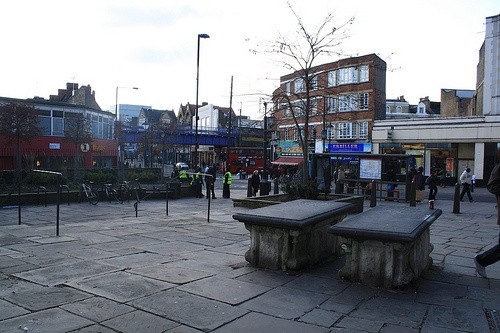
[271,157,303,166]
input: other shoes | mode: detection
[473,255,487,278]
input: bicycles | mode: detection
[103,179,147,204]
[77,180,101,205]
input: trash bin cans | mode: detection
[259,179,271,196]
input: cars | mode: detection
[176,162,188,169]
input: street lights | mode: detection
[193,33,212,169]
[115,85,139,120]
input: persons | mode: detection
[459,167,475,203]
[362,182,373,201]
[323,166,357,192]
[387,171,398,197]
[205,165,217,199]
[223,168,231,199]
[473,149,500,278]
[175,165,204,198]
[270,165,287,180]
[413,167,446,201]
[251,170,260,197]
